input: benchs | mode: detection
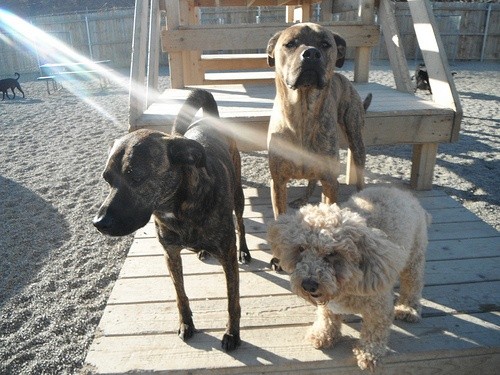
[36,71,107,94]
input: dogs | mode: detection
[0,72,25,101]
[267,186,432,373]
[266,19,373,272]
[411,63,458,96]
[91,88,252,352]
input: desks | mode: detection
[40,60,111,90]
